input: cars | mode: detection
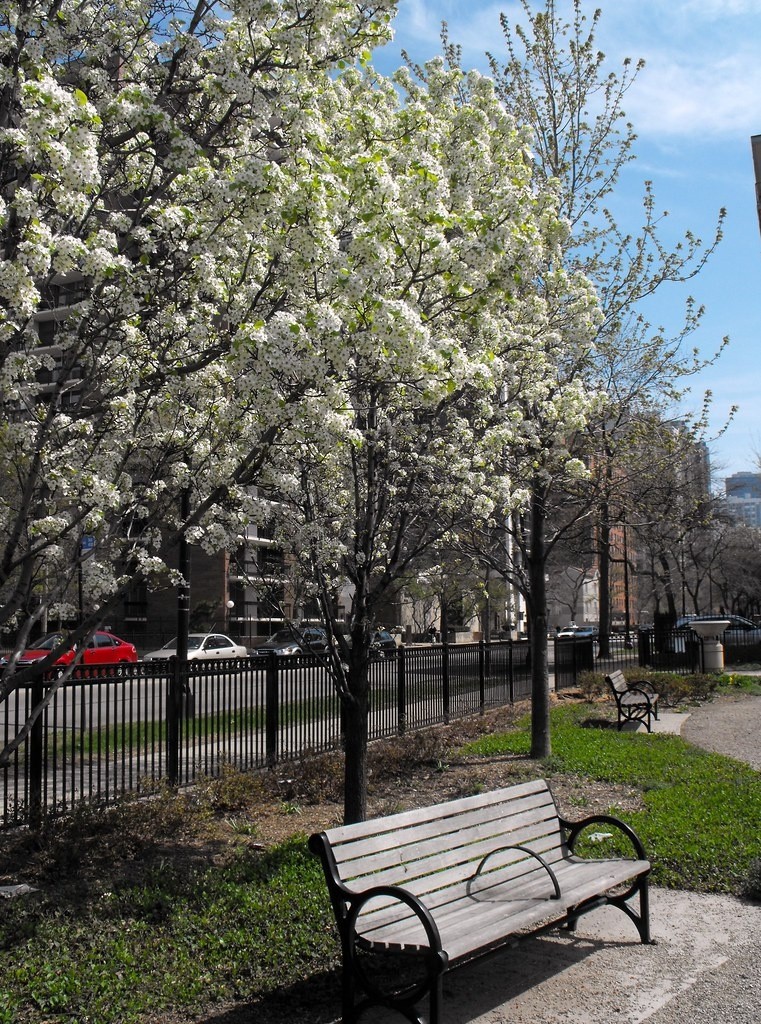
[0,630,138,681]
[557,626,579,638]
[335,631,396,659]
[574,626,598,637]
[143,633,247,672]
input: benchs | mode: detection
[605,669,660,733]
[307,779,658,1024]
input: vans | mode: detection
[670,614,761,653]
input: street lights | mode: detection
[594,520,657,650]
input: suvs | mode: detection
[249,627,327,667]
